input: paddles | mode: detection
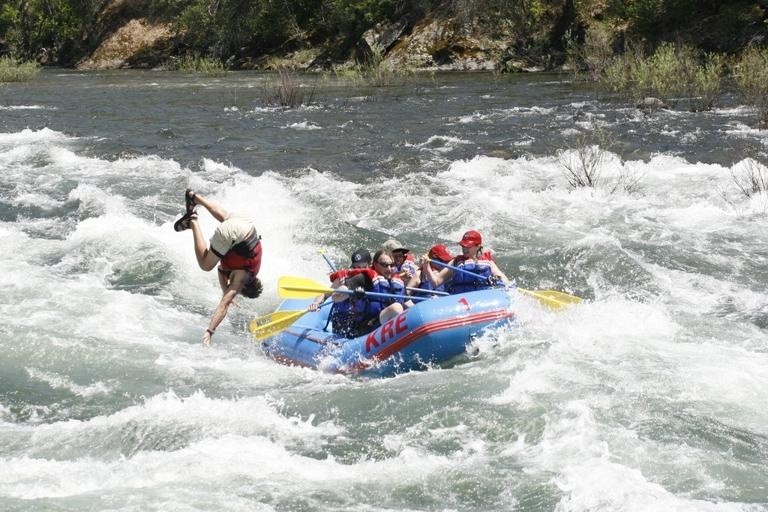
[277,277,432,301]
[426,258,583,310]
[249,300,334,341]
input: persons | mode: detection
[305,228,512,336]
[173,188,264,346]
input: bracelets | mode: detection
[206,328,215,335]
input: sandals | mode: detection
[174,212,197,231]
[185,189,196,213]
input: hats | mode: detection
[459,231,481,248]
[429,245,452,261]
[383,239,409,253]
[351,249,371,267]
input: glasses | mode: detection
[379,262,394,267]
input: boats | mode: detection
[261,283,518,383]
